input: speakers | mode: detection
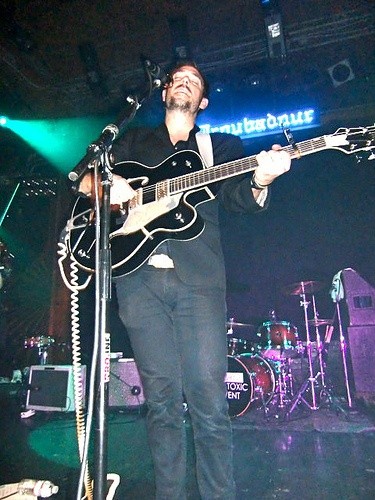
[341,271,375,326]
[108,361,145,409]
[26,365,86,411]
[344,327,375,398]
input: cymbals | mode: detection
[226,321,256,330]
[302,318,330,325]
[280,282,326,295]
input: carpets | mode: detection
[229,404,375,434]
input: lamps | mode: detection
[206,59,357,100]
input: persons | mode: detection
[78,62,291,500]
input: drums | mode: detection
[224,353,276,417]
[226,338,258,356]
[258,322,299,362]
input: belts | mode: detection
[148,255,174,269]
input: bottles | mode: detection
[40,347,49,366]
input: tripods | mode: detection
[261,286,349,418]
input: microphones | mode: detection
[146,60,172,88]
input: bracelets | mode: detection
[251,175,268,190]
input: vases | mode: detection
[39,347,49,365]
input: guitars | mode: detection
[68,122,375,278]
[0,335,56,349]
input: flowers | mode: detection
[24,335,55,348]
